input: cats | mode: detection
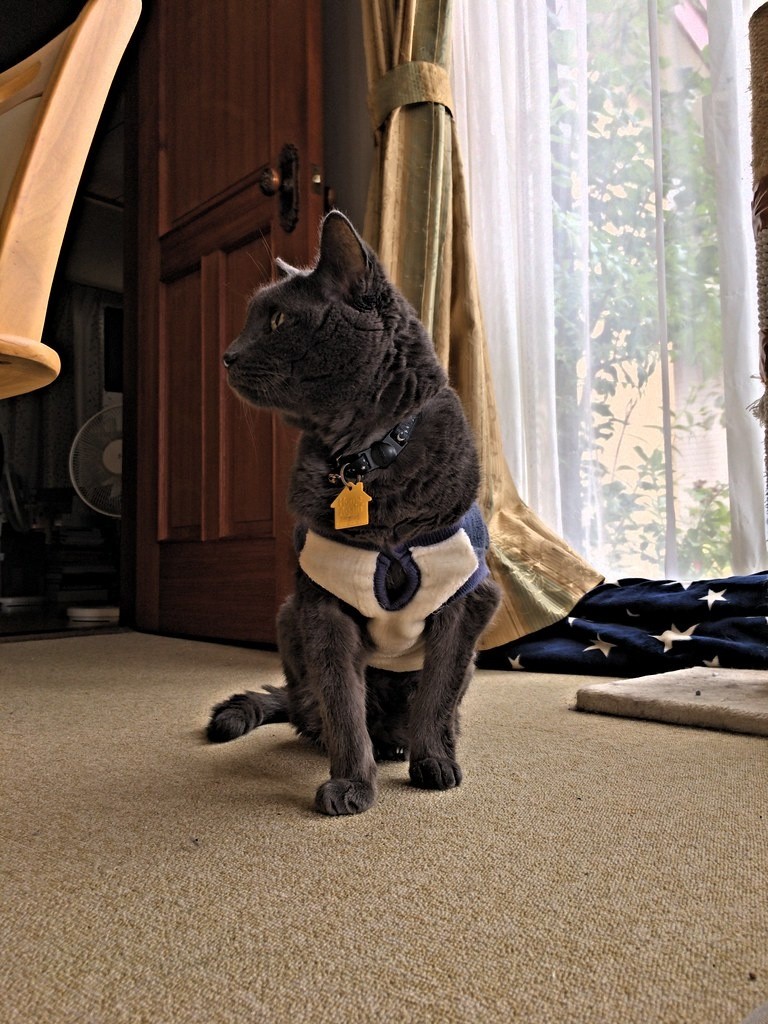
[202,207,501,820]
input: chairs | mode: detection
[0,1,145,403]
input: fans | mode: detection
[67,404,126,624]
